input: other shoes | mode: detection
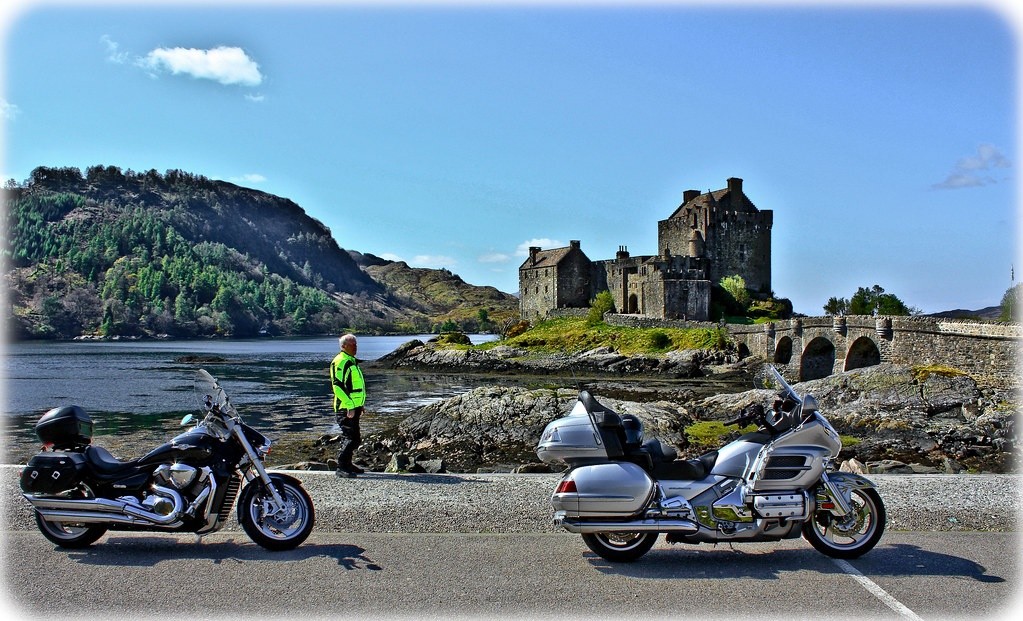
[352,463,365,474]
[337,464,357,478]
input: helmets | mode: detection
[619,414,643,450]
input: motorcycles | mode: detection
[20,368,316,551]
[534,361,885,566]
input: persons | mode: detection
[330,333,366,479]
